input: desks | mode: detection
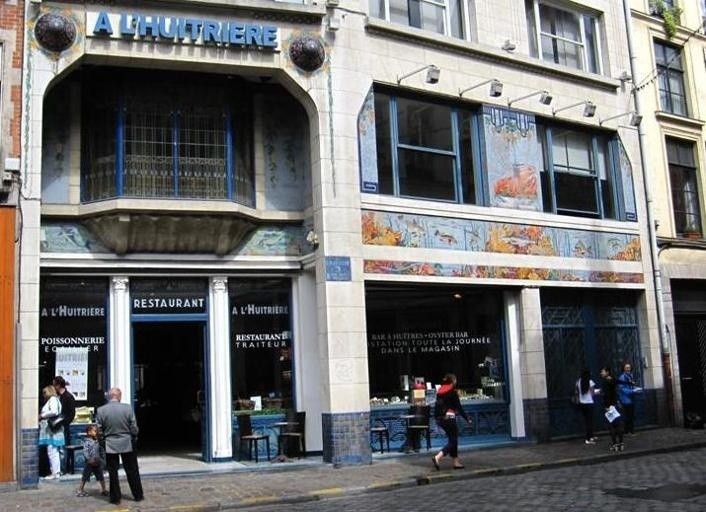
[266,422,300,462]
[399,414,425,449]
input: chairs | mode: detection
[407,405,431,451]
[64,431,88,474]
[281,411,306,459]
[370,416,389,454]
[237,413,270,463]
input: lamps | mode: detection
[599,111,643,126]
[460,77,503,97]
[554,100,596,117]
[397,65,440,84]
[509,88,553,105]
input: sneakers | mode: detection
[100,489,110,496]
[45,473,61,482]
[75,489,92,498]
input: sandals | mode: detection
[585,437,600,445]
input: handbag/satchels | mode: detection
[47,413,66,432]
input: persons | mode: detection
[618,364,641,436]
[595,368,636,452]
[75,424,111,498]
[47,376,77,475]
[94,387,144,504]
[37,385,67,480]
[431,373,472,471]
[574,364,605,445]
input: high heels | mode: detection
[608,442,625,453]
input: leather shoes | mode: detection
[453,465,466,470]
[430,456,441,472]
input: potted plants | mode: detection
[231,407,287,461]
